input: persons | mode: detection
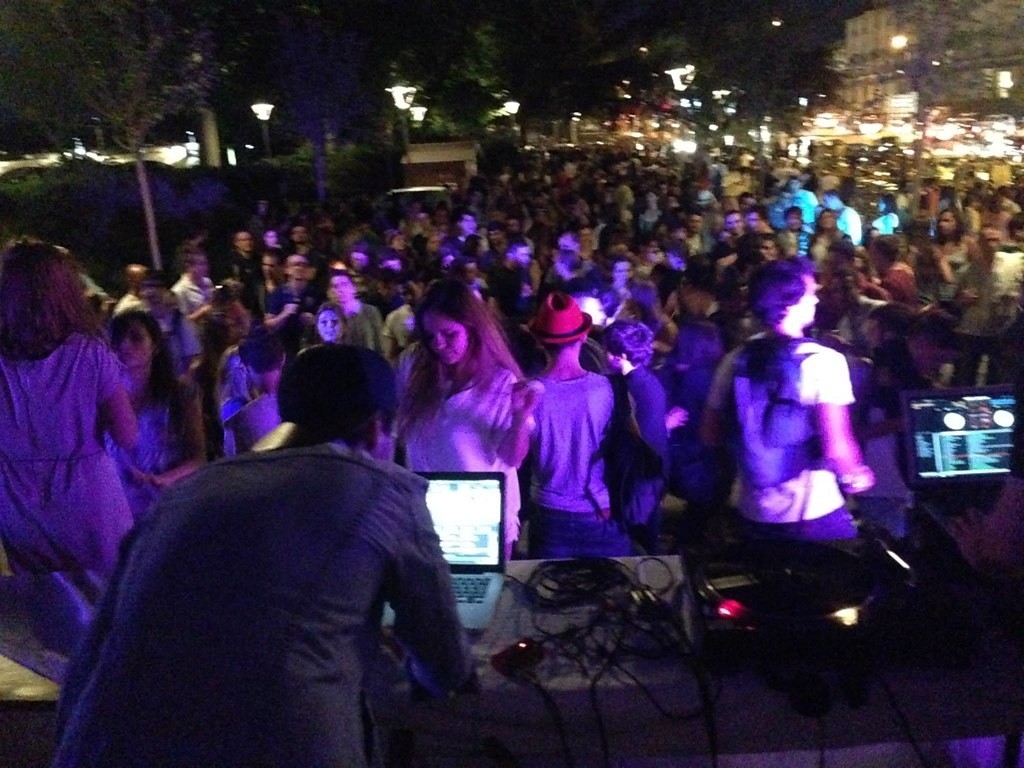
[497,291,643,558]
[0,117,1023,657]
[46,340,484,768]
[702,258,874,543]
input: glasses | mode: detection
[117,329,152,341]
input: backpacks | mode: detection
[726,337,820,489]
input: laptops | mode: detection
[900,382,1024,546]
[378,473,507,631]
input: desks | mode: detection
[368,555,697,768]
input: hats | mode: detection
[692,189,714,205]
[277,341,396,428]
[820,175,842,190]
[528,291,592,343]
[287,254,308,265]
